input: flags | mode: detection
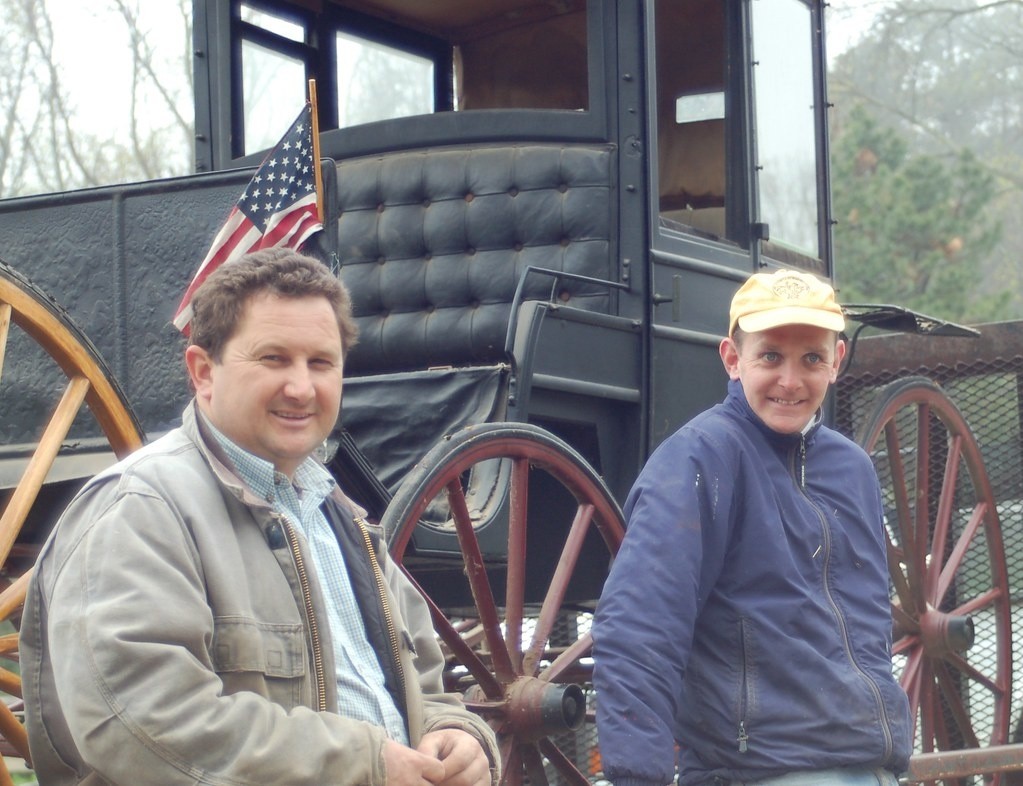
[173,103,323,340]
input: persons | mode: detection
[587,268,912,785]
[18,247,503,785]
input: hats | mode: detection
[728,269,846,339]
